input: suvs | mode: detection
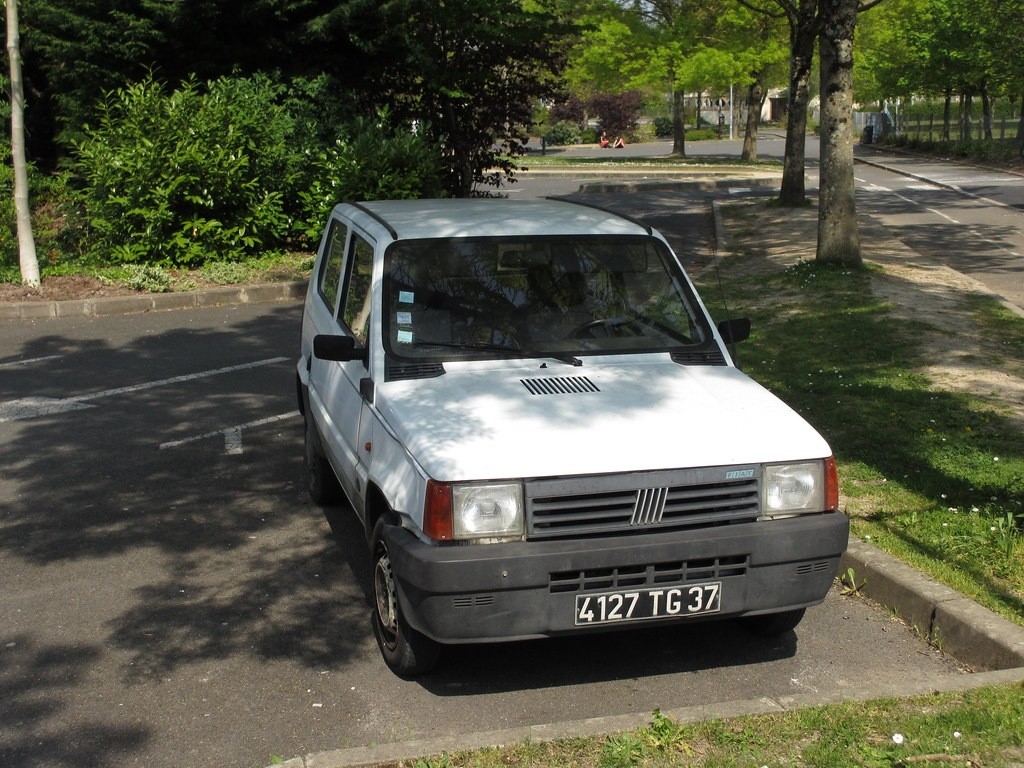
[296,199,851,680]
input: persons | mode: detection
[599,132,609,149]
[613,135,625,148]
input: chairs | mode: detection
[391,262,485,346]
[506,261,612,344]
